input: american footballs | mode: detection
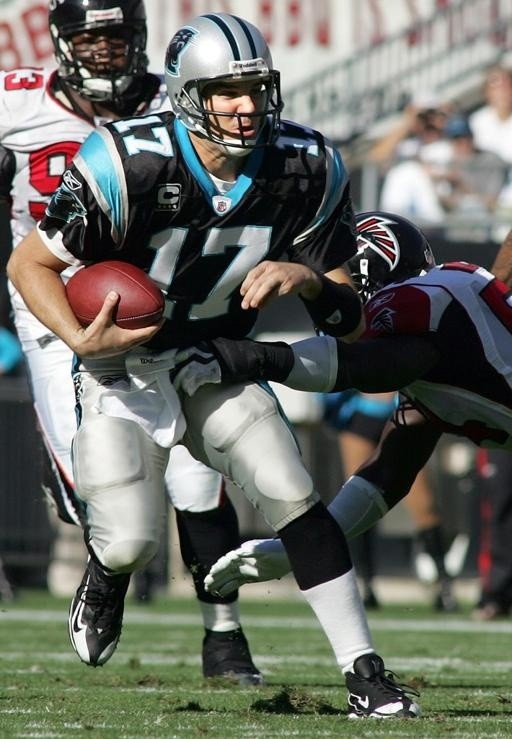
[66,262,166,329]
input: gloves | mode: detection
[171,333,259,397]
[203,536,293,598]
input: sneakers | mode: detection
[202,642,261,686]
[67,553,130,667]
[343,653,420,720]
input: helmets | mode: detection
[47,0,150,109]
[163,12,283,151]
[346,211,436,305]
